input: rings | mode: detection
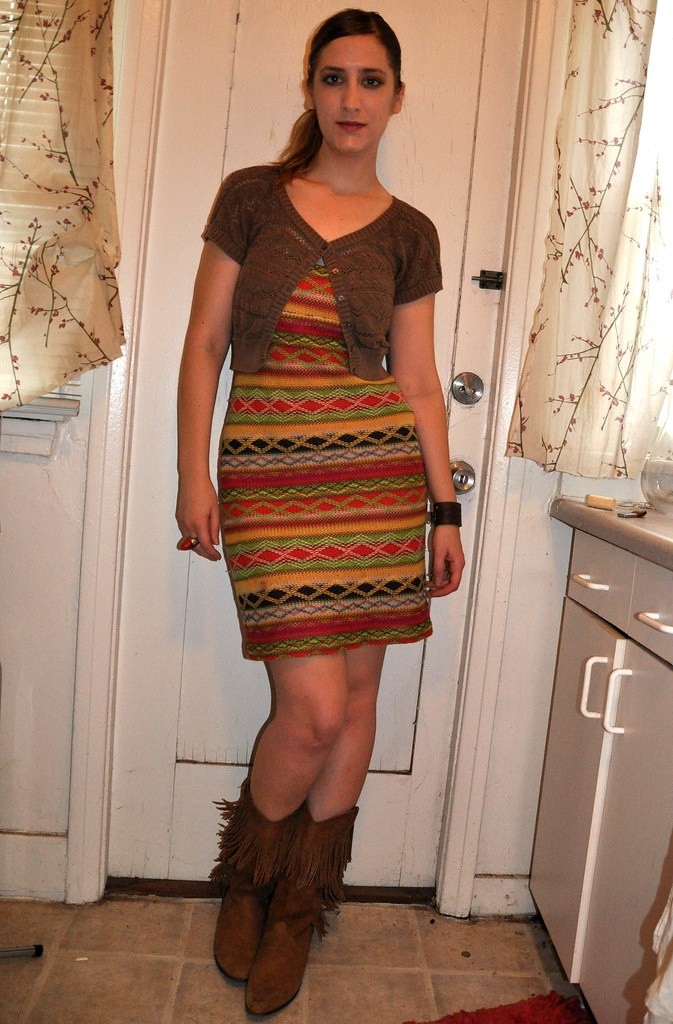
[180,537,198,551]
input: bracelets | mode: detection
[433,501,462,527]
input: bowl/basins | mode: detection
[641,456,673,513]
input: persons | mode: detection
[177,7,465,1018]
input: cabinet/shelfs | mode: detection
[529,526,673,1024]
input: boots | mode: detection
[211,779,305,982]
[245,799,359,1016]
